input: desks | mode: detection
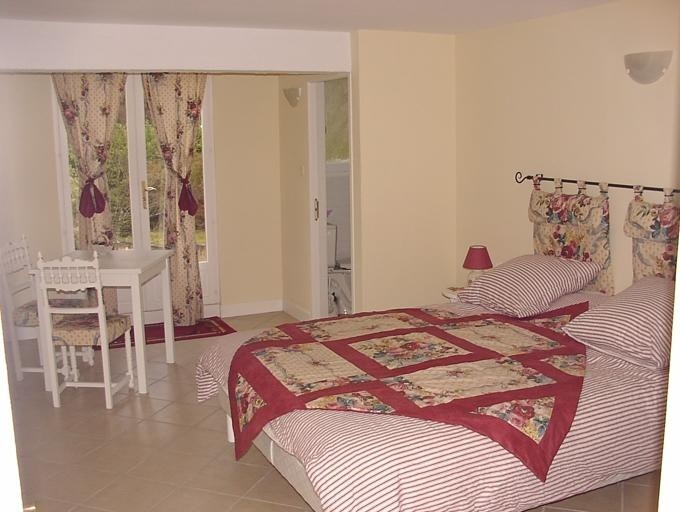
[27,247,177,395]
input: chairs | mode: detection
[36,249,135,410]
[0,233,95,381]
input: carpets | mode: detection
[92,316,238,351]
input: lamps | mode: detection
[283,87,303,108]
[461,245,494,286]
[624,50,674,85]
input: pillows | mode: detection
[450,254,603,318]
[558,274,675,371]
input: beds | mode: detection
[192,285,676,512]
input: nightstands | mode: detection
[441,285,466,303]
